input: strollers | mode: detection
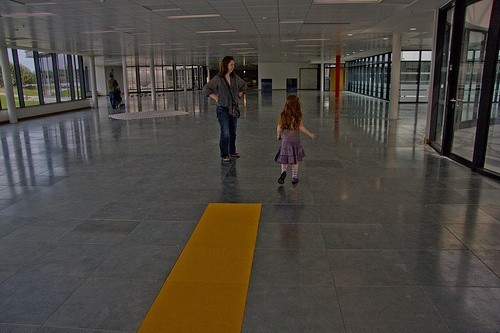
[114,88,125,108]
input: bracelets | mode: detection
[237,95,241,99]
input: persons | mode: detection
[273,94,319,184]
[107,73,123,110]
[203,56,248,161]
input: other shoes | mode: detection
[278,170,286,184]
[223,156,232,162]
[230,153,240,158]
[292,177,299,184]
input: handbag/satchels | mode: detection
[233,103,240,118]
